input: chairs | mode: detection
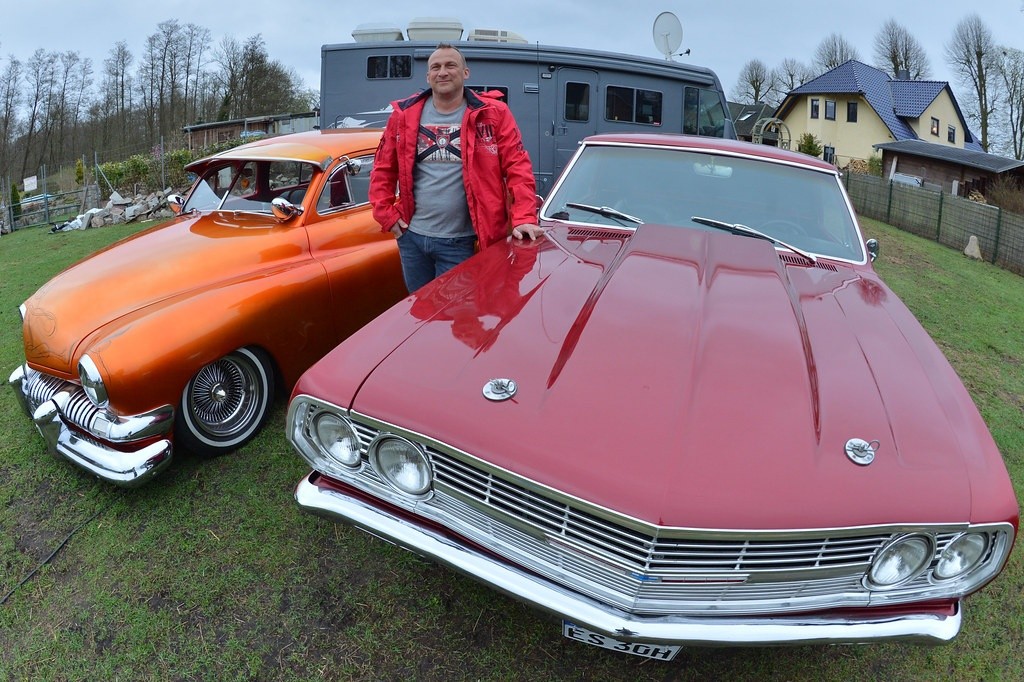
[277,188,333,210]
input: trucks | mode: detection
[319,15,742,208]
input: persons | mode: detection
[369,41,545,294]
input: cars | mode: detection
[8,128,407,488]
[284,134,1021,663]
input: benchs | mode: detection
[339,178,371,205]
